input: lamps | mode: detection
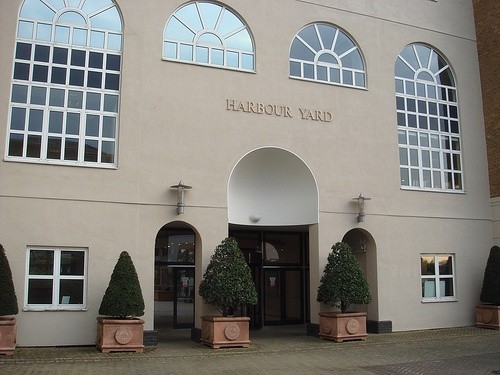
[352,193,372,222]
[170,180,192,215]
[250,213,260,222]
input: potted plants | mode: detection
[317,241,373,342]
[0,244,19,356]
[475,246,500,329]
[96,251,145,353]
[198,237,258,350]
